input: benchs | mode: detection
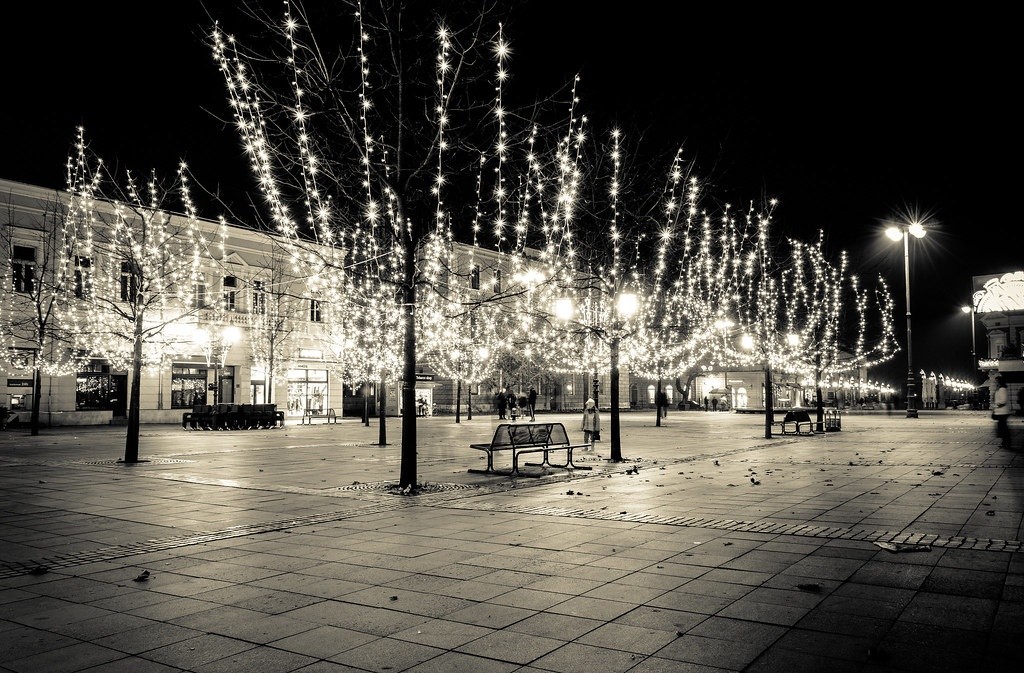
[301,408,341,424]
[466,421,594,479]
[769,410,826,438]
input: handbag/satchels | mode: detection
[593,432,600,441]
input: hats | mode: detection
[585,398,595,404]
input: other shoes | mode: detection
[586,450,595,454]
[512,418,516,421]
[582,448,588,452]
[530,420,535,423]
[499,417,506,419]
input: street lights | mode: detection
[884,221,926,420]
[961,305,978,394]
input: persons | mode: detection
[704,397,709,411]
[498,390,517,420]
[418,395,428,418]
[991,376,1012,450]
[660,388,668,418]
[712,397,718,411]
[528,387,536,422]
[581,399,601,451]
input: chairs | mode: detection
[182,403,287,431]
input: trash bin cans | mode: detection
[825,410,841,431]
